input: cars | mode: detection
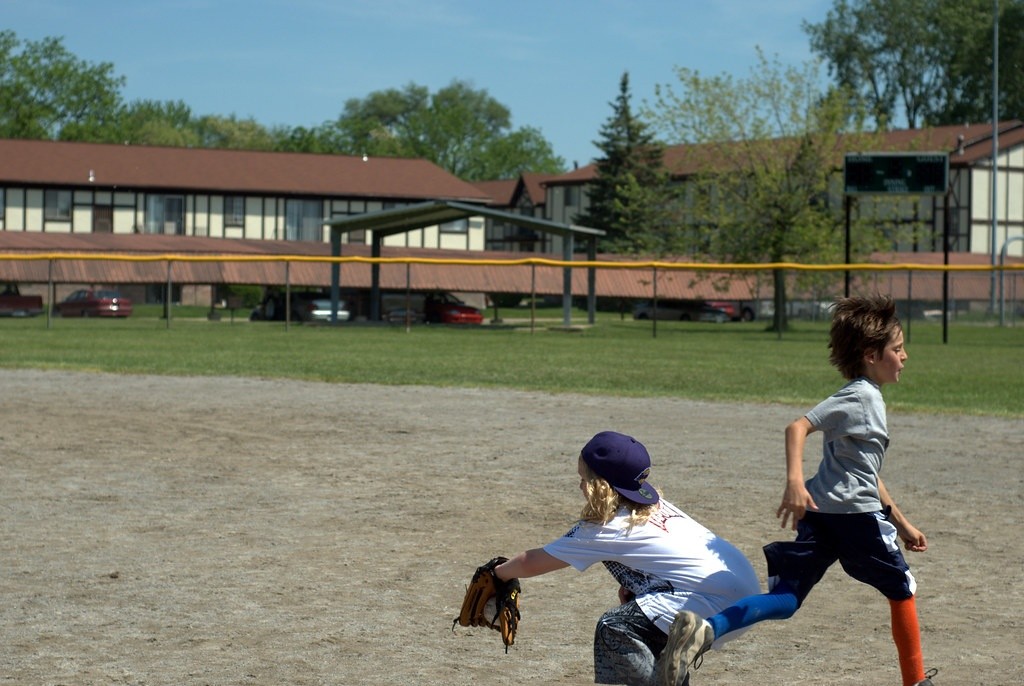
[0,291,44,317]
[250,292,350,322]
[435,293,484,324]
[54,288,134,319]
[634,298,730,322]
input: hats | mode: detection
[581,431,660,506]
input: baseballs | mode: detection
[483,595,506,627]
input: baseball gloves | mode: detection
[456,556,523,649]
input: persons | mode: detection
[659,294,938,685]
[457,431,762,686]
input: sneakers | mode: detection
[661,610,714,684]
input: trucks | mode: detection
[729,301,775,322]
